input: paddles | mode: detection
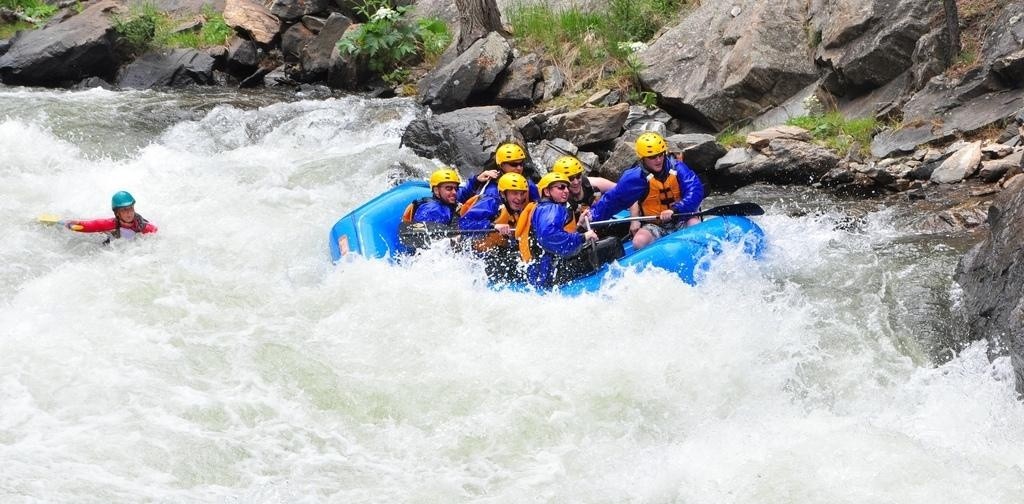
[399,220,517,249]
[584,215,600,268]
[588,203,765,225]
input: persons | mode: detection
[484,143,539,202]
[63,190,156,242]
[528,171,598,281]
[458,172,533,256]
[409,169,498,259]
[550,158,641,240]
[586,132,702,249]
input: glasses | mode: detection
[502,160,524,167]
[645,152,664,160]
[549,184,569,190]
[569,174,581,182]
[437,186,461,192]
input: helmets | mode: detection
[112,191,136,215]
[497,172,529,194]
[553,156,584,177]
[537,172,571,198]
[496,143,526,167]
[636,134,668,161]
[430,168,460,189]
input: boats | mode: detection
[329,181,764,298]
